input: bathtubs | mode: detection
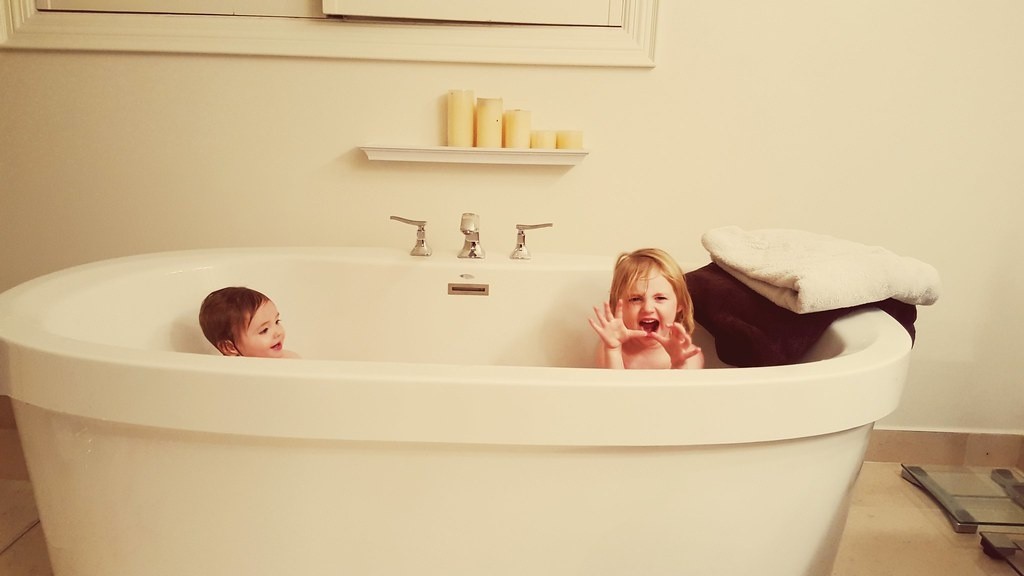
[1,244,914,575]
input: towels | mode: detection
[682,225,943,366]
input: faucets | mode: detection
[458,213,485,260]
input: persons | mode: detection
[588,247,704,369]
[199,287,300,359]
